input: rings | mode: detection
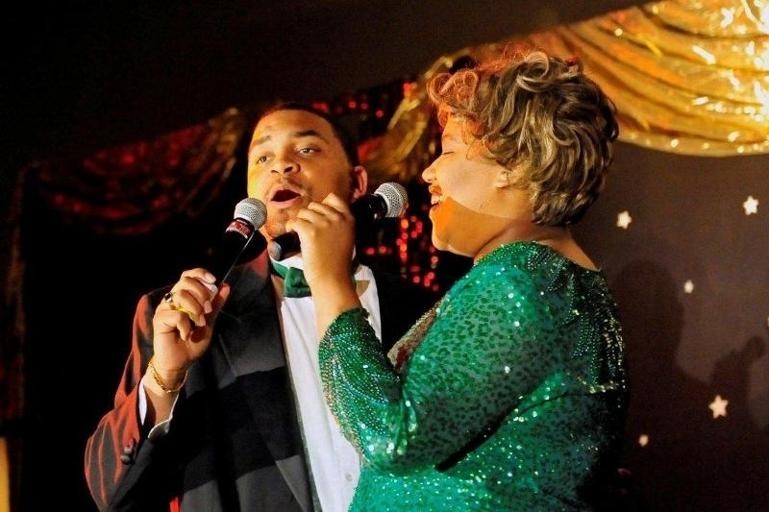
[163,291,172,300]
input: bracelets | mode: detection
[152,357,191,393]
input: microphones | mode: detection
[267,181,409,261]
[178,197,268,328]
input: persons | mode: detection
[287,41,632,510]
[81,100,450,511]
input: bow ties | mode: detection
[282,266,356,298]
